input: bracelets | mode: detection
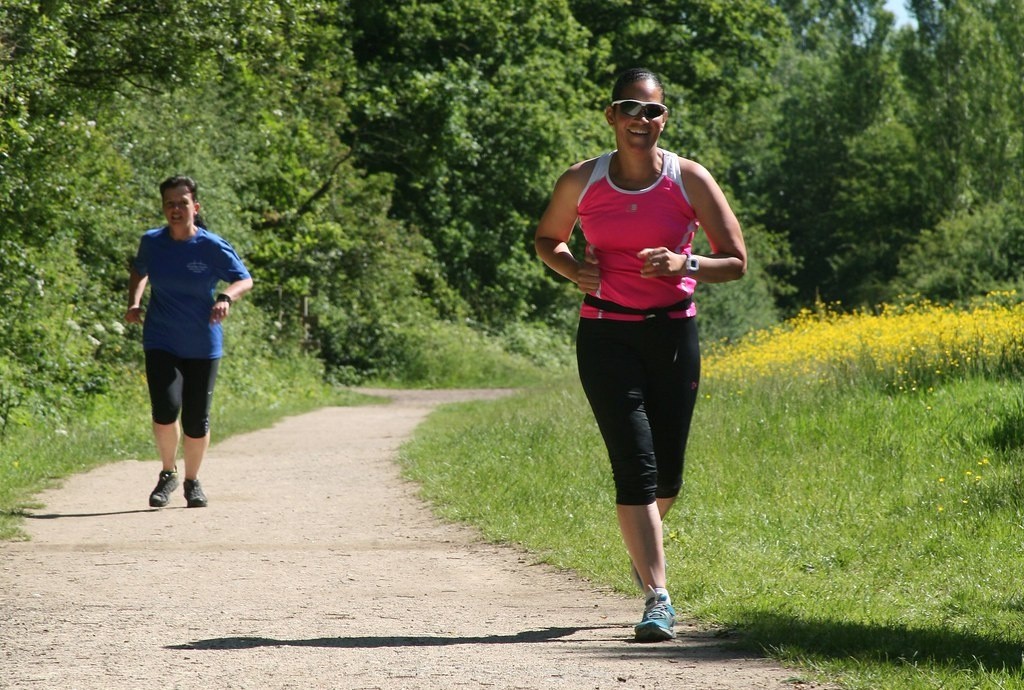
[217,294,232,304]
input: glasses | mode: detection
[611,100,668,118]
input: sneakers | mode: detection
[633,563,644,588]
[183,478,208,507]
[150,465,179,507]
[635,584,676,640]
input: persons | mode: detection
[534,69,746,640]
[126,176,253,507]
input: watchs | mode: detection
[685,253,699,276]
